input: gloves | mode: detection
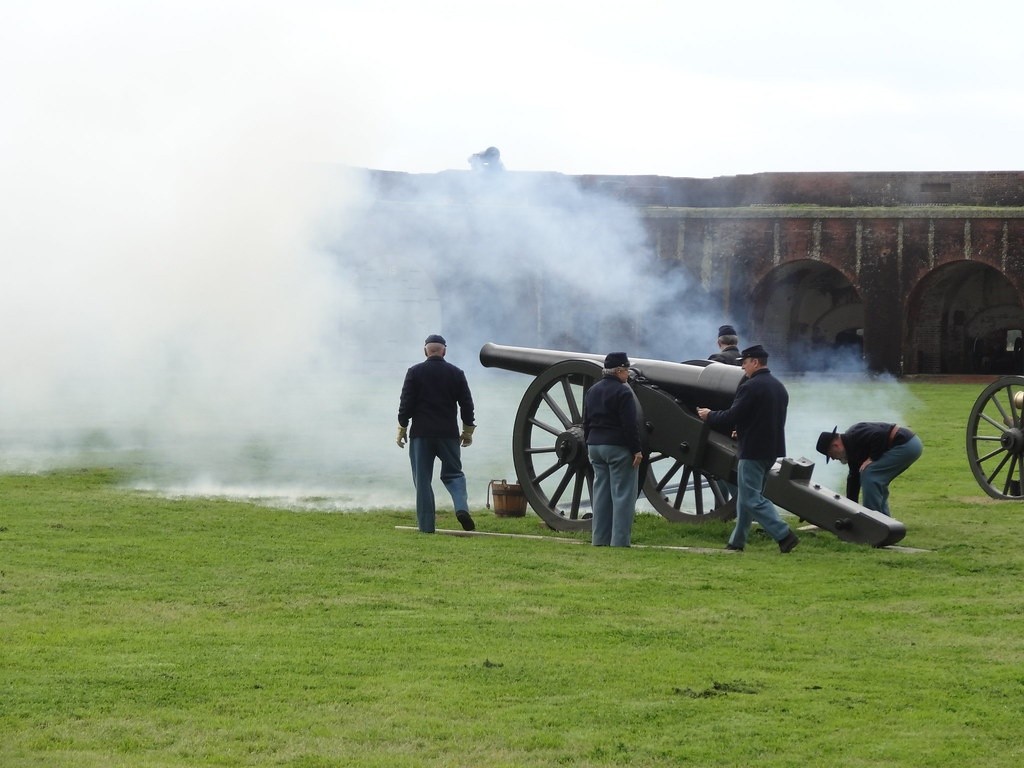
[395,426,407,449]
[460,423,477,447]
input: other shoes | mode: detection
[456,511,474,531]
[779,529,797,553]
[724,544,743,551]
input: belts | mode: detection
[888,424,901,445]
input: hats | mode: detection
[604,352,630,369]
[735,345,768,362]
[816,425,837,464]
[424,334,446,348]
[718,325,736,337]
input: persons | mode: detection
[697,345,801,553]
[395,335,477,533]
[816,422,922,517]
[707,326,741,366]
[582,352,642,547]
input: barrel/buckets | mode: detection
[489,479,528,517]
